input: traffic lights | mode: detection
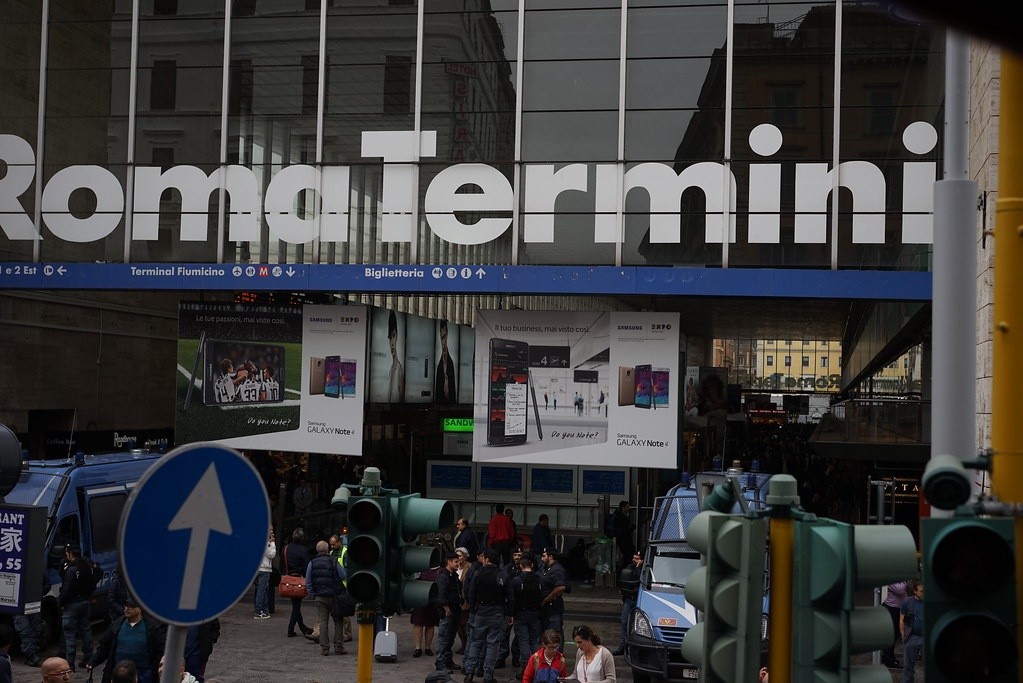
[920,518,1019,683]
[811,518,919,683]
[389,493,455,615]
[681,510,767,683]
[347,494,389,610]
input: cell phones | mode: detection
[618,365,670,410]
[184,330,286,411]
[310,356,357,400]
[487,337,543,447]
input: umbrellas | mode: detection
[86,667,93,683]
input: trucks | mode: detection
[623,454,774,683]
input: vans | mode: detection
[4,449,166,628]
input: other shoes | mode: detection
[889,660,903,670]
[335,649,347,655]
[343,636,352,642]
[412,648,422,658]
[288,632,297,637]
[304,633,320,643]
[302,628,314,635]
[425,648,433,656]
[613,645,627,655]
[438,646,521,683]
[321,650,329,656]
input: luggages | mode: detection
[374,614,398,661]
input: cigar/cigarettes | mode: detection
[272,534,274,535]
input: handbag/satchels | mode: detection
[331,596,356,618]
[278,575,307,597]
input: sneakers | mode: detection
[254,613,270,619]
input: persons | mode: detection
[436,321,456,403]
[685,375,726,416]
[249,450,363,540]
[305,541,348,655]
[613,551,645,656]
[607,500,634,568]
[279,528,314,638]
[253,525,277,619]
[882,582,924,683]
[562,625,617,683]
[304,534,352,643]
[760,667,768,683]
[410,505,597,683]
[544,390,604,416]
[388,310,404,402]
[724,423,839,516]
[215,359,279,403]
[0,542,221,683]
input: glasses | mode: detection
[47,670,73,677]
[124,604,133,610]
[545,644,560,649]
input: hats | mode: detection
[513,546,524,554]
[425,671,451,683]
[126,597,138,606]
[543,547,558,553]
